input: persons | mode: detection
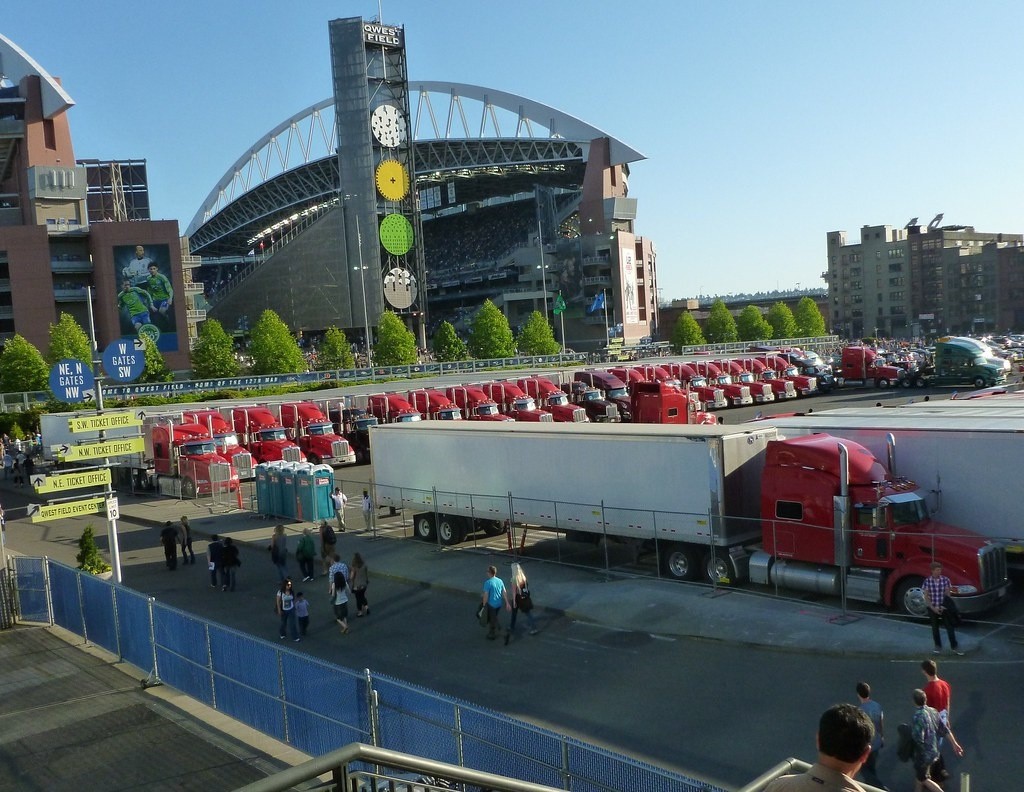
[897,660,964,792]
[270,525,290,581]
[157,515,196,571]
[508,563,539,635]
[327,551,371,634]
[299,335,434,371]
[319,518,338,575]
[295,528,317,582]
[117,245,175,335]
[361,488,371,532]
[762,703,876,792]
[0,432,41,489]
[206,534,242,592]
[329,487,348,532]
[477,565,510,647]
[854,681,888,791]
[421,194,573,270]
[921,561,965,657]
[275,577,311,643]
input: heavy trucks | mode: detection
[121,406,262,482]
[207,403,307,468]
[166,398,356,467]
[273,324,1024,459]
[367,420,1010,623]
[39,408,239,500]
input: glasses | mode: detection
[285,583,292,586]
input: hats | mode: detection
[362,487,368,491]
[165,521,173,526]
[931,561,942,569]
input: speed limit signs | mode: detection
[105,496,120,521]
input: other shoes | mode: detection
[356,612,364,617]
[486,634,496,640]
[280,635,286,639]
[222,585,226,592]
[341,626,349,634]
[528,628,538,634]
[365,607,370,614]
[507,626,514,631]
[933,646,941,654]
[292,637,301,642]
[211,583,215,588]
[504,634,510,646]
[952,648,964,656]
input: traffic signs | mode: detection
[55,437,146,463]
[99,338,147,383]
[70,407,147,434]
[51,358,96,406]
[26,497,107,524]
[30,469,111,493]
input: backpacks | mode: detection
[322,525,337,546]
[517,580,534,615]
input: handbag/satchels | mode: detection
[274,589,283,614]
[236,557,242,568]
[329,597,335,607]
[296,538,310,562]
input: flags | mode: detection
[554,294,566,315]
[588,293,605,312]
